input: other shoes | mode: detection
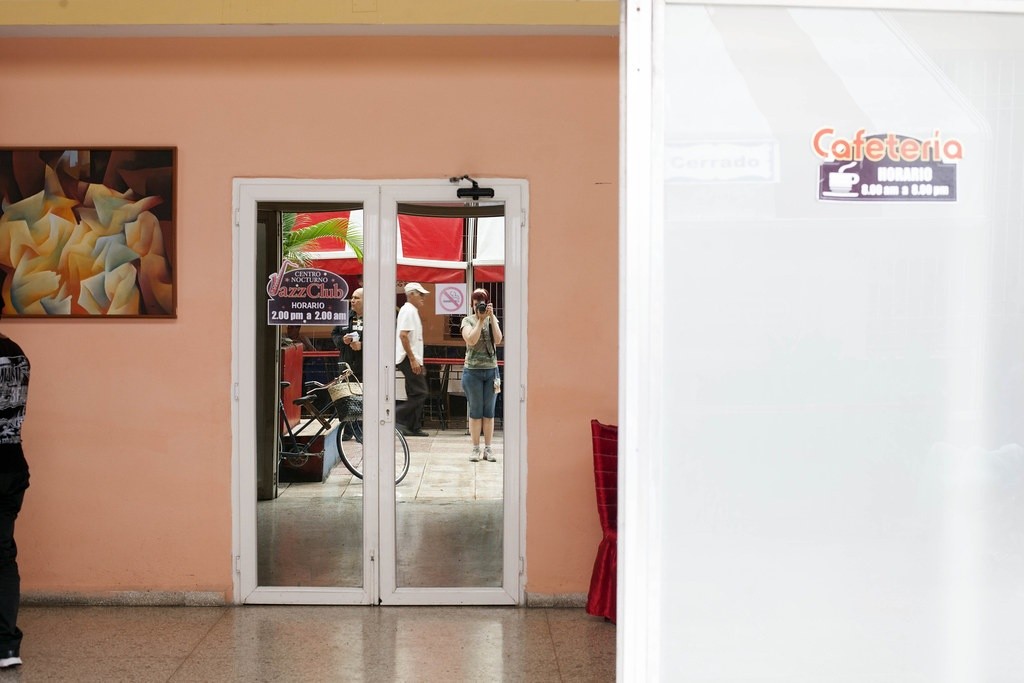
[403,429,430,436]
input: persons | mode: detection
[395,282,430,437]
[281,325,316,415]
[331,288,363,444]
[461,288,502,462]
[0,264,30,669]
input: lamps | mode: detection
[450,176,495,201]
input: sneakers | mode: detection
[483,449,496,462]
[470,449,481,461]
[0,649,23,668]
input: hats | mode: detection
[404,283,430,294]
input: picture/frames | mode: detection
[0,142,180,323]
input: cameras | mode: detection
[476,303,488,314]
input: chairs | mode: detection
[585,419,618,625]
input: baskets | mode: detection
[329,372,363,422]
